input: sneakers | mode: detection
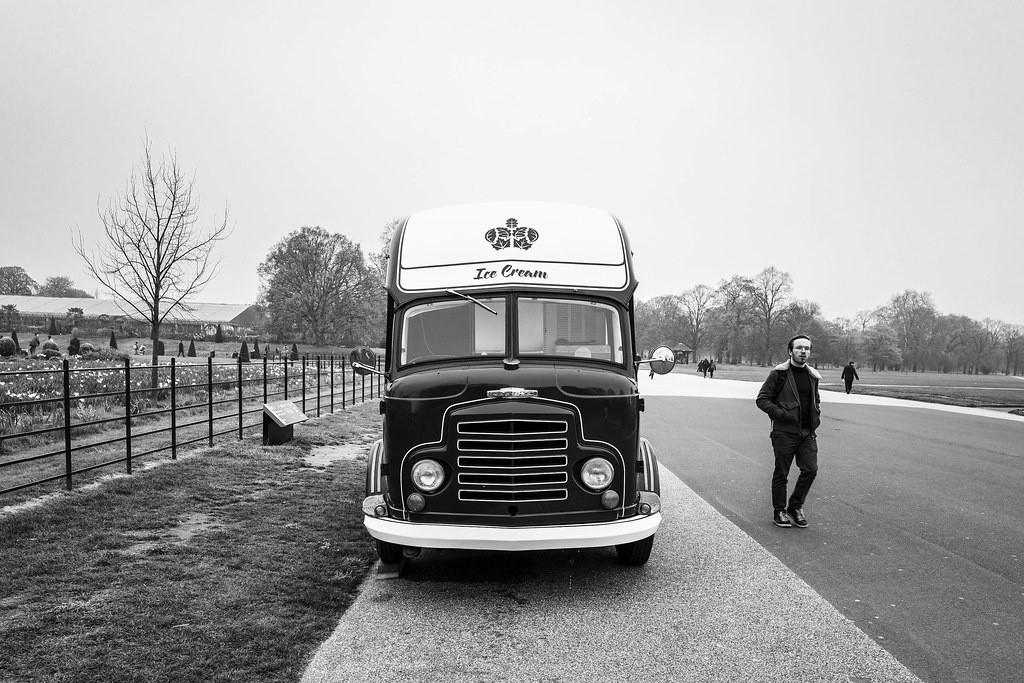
[787,506,808,528]
[773,510,791,527]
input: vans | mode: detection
[349,204,676,568]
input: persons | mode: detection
[697,360,703,372]
[142,345,146,355]
[177,340,185,357]
[134,342,139,355]
[841,361,859,394]
[232,351,238,358]
[709,360,716,378]
[48,336,53,341]
[265,345,270,354]
[210,348,215,358]
[756,334,822,527]
[275,345,289,356]
[702,358,709,378]
[30,333,40,354]
